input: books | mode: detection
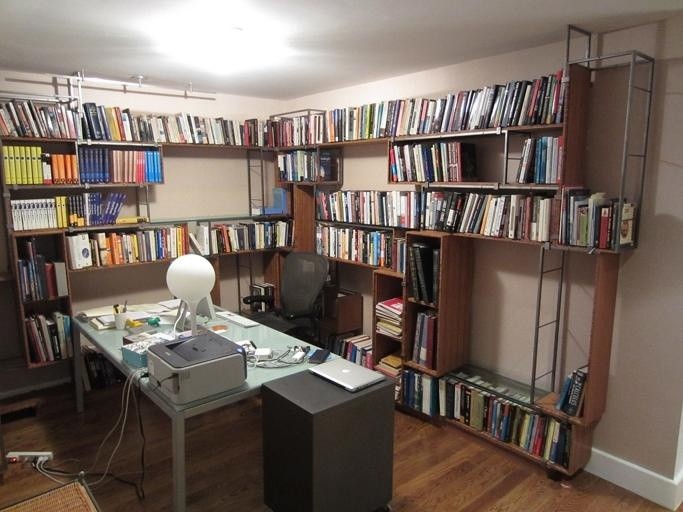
[211,220,295,253]
[266,70,564,148]
[65,222,209,270]
[421,191,556,242]
[375,352,402,400]
[412,311,437,369]
[408,242,440,306]
[252,188,287,215]
[342,335,372,369]
[3,145,162,185]
[557,369,588,416]
[80,345,125,390]
[403,370,571,469]
[316,190,420,228]
[17,238,68,301]
[516,137,562,184]
[560,187,635,249]
[376,297,403,339]
[316,227,406,273]
[11,193,147,231]
[278,149,337,183]
[1,98,267,146]
[25,311,73,362]
[390,141,477,182]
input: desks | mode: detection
[71,300,341,512]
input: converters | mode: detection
[255,348,273,359]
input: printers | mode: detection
[147,330,247,405]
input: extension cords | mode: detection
[5,452,53,463]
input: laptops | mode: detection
[308,358,385,392]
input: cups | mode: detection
[114,312,127,330]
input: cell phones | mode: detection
[309,350,331,363]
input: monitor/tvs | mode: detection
[175,294,216,334]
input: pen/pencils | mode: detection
[113,300,128,313]
[250,341,257,349]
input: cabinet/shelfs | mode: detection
[0,48,656,481]
[260,356,396,511]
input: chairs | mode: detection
[243,253,330,347]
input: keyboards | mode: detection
[216,311,260,327]
